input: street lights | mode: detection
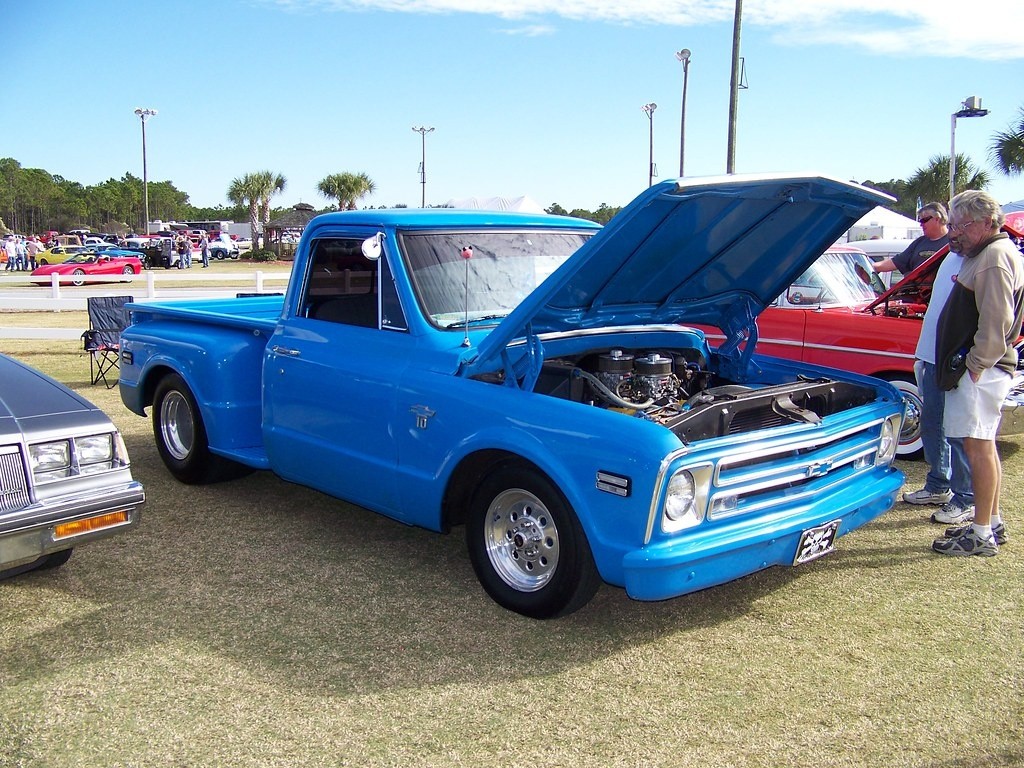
[949,93,990,201]
[674,48,692,178]
[642,101,658,189]
[134,107,157,235]
[410,125,436,210]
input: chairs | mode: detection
[308,295,367,327]
[84,257,94,264]
[79,296,134,390]
[53,249,60,254]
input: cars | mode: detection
[3,230,123,247]
[34,245,111,267]
[85,243,146,266]
[237,237,253,249]
[0,354,147,574]
[672,209,1024,460]
[839,238,922,292]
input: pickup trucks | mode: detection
[118,171,908,625]
[119,229,239,270]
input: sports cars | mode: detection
[29,249,143,287]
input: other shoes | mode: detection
[188,266,191,268]
[5,268,35,272]
[201,265,209,268]
[178,268,185,269]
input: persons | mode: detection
[171,234,193,269]
[121,234,127,247]
[5,236,54,272]
[872,203,950,292]
[902,212,975,523]
[198,234,211,267]
[932,191,1024,556]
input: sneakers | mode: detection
[944,523,1007,545]
[932,524,998,558]
[900,487,955,506]
[930,502,975,525]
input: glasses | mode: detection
[947,223,952,230]
[919,213,938,223]
[951,215,990,231]
